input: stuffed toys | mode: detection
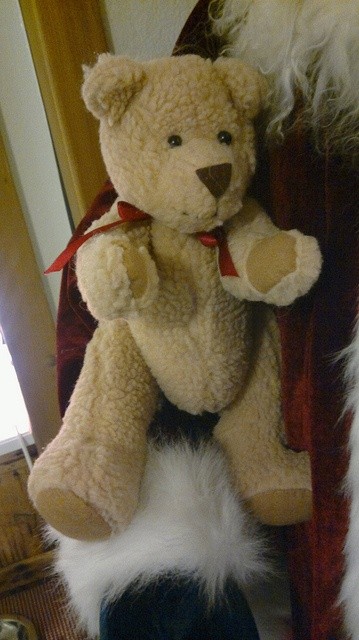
[26,53,323,542]
